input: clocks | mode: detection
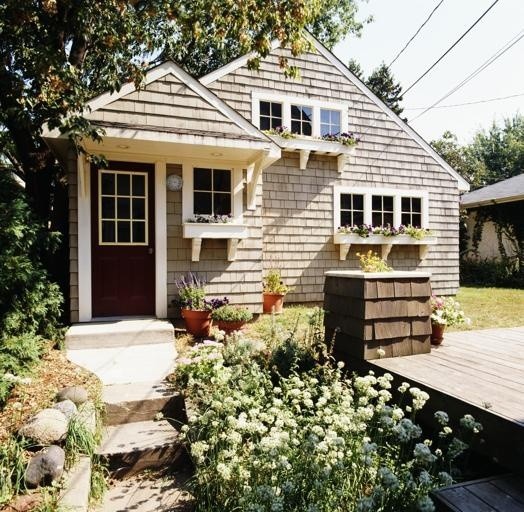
[166,174,183,192]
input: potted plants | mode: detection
[262,269,288,313]
[216,306,253,335]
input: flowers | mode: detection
[174,272,229,310]
[430,294,470,325]
[338,223,432,240]
[261,126,360,146]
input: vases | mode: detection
[181,309,213,338]
[430,325,447,346]
